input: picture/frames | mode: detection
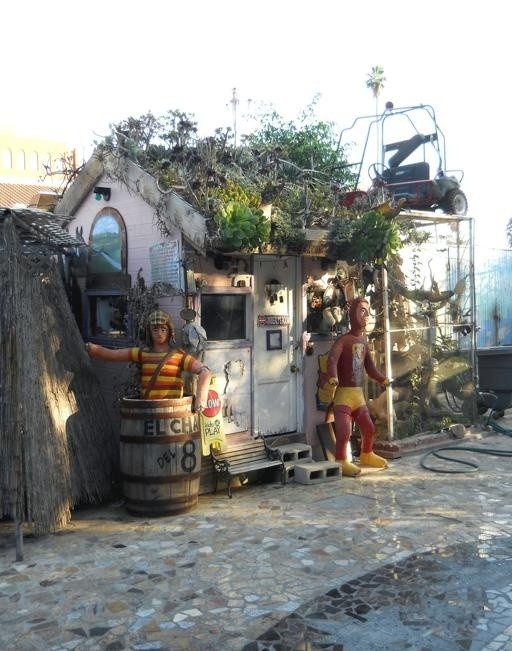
[266,330,282,350]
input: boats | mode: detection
[442,344,512,416]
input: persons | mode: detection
[86,309,211,414]
[327,297,390,478]
